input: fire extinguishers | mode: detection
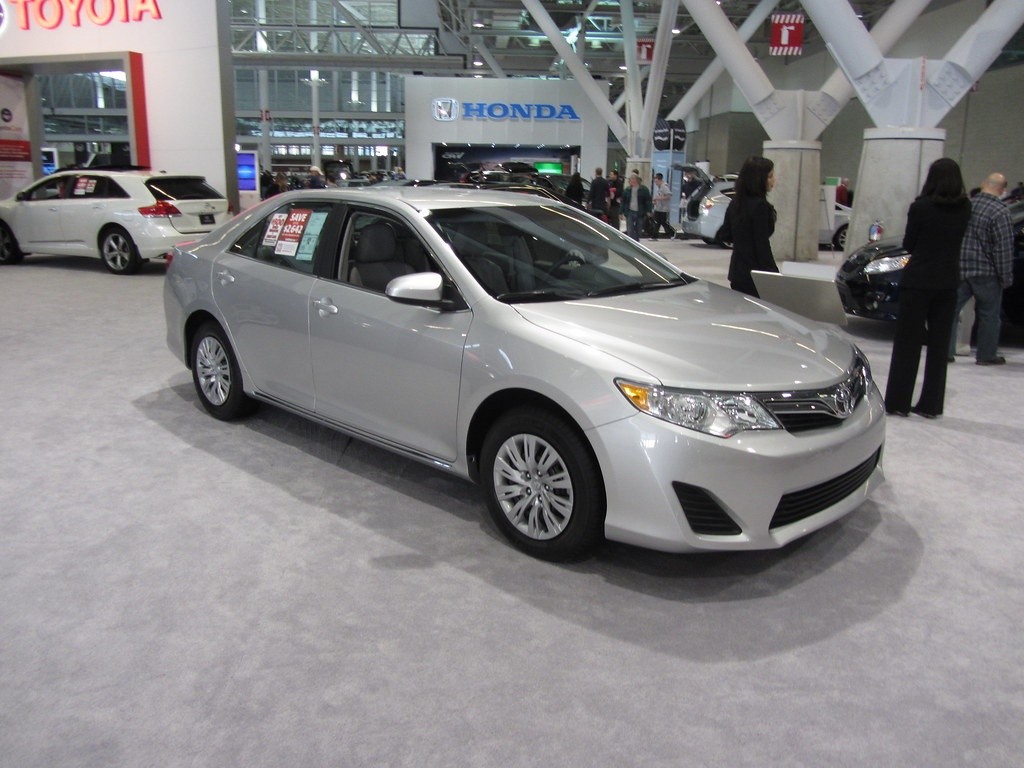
[868,217,885,243]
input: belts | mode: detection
[629,209,640,213]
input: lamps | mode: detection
[471,9,483,78]
[591,40,603,49]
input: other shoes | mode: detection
[975,356,1006,366]
[888,408,909,418]
[948,357,955,363]
[910,407,939,420]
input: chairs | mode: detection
[435,221,512,304]
[348,221,417,294]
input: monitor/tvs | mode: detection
[534,162,563,174]
[41,151,55,175]
[237,153,256,191]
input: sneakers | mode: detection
[648,237,659,241]
[671,229,677,239]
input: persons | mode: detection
[681,172,701,222]
[511,175,609,267]
[836,177,852,208]
[260,166,327,198]
[884,158,972,419]
[392,165,405,181]
[947,172,1015,366]
[567,168,676,242]
[723,156,779,300]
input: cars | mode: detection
[833,180,1024,323]
[157,176,891,568]
[680,169,855,251]
[0,159,235,278]
